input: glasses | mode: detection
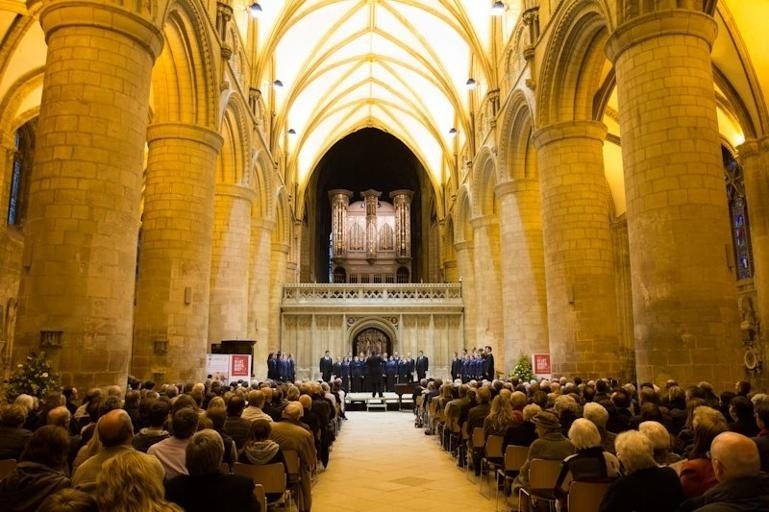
[704,450,727,472]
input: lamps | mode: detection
[288,128,295,135]
[248,1,260,16]
[273,80,282,88]
[449,128,459,136]
[466,79,477,87]
[491,0,504,13]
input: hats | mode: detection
[530,411,562,430]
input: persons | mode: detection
[238,379,243,383]
[523,381,530,390]
[316,379,323,383]
[484,346,494,381]
[84,386,104,398]
[251,380,260,389]
[296,394,328,469]
[406,352,414,382]
[382,352,388,362]
[341,356,350,393]
[127,375,142,391]
[471,347,476,354]
[443,385,468,450]
[587,380,595,388]
[576,381,587,394]
[366,351,387,398]
[457,387,491,467]
[637,381,655,391]
[164,429,261,512]
[0,425,71,512]
[36,487,97,512]
[551,382,560,393]
[716,392,737,410]
[14,393,33,418]
[638,388,659,403]
[282,382,291,391]
[510,378,519,386]
[598,400,616,426]
[437,385,455,445]
[696,431,769,512]
[351,356,361,392]
[229,381,238,388]
[678,398,717,442]
[166,383,180,399]
[412,380,427,414]
[435,377,443,385]
[191,383,205,406]
[207,374,213,382]
[210,382,219,387]
[451,351,459,382]
[393,352,401,362]
[478,348,485,380]
[497,404,542,498]
[161,383,170,391]
[35,392,66,433]
[321,382,342,425]
[735,380,751,396]
[504,382,514,391]
[302,379,307,383]
[242,381,248,388]
[359,351,365,364]
[669,406,687,428]
[287,353,295,383]
[294,380,301,388]
[277,352,284,381]
[451,389,478,457]
[333,356,342,377]
[311,383,336,441]
[146,406,200,481]
[533,390,548,409]
[0,403,33,460]
[260,383,269,389]
[539,379,551,392]
[333,380,340,422]
[329,382,348,420]
[302,381,312,393]
[499,388,513,400]
[159,394,172,409]
[277,380,282,385]
[492,378,501,388]
[223,391,236,406]
[573,376,582,387]
[582,384,597,401]
[610,378,619,388]
[223,384,233,393]
[519,378,523,385]
[72,408,166,494]
[282,352,288,378]
[333,377,345,420]
[463,354,469,381]
[752,392,769,473]
[172,383,183,394]
[460,348,467,380]
[204,408,238,465]
[165,394,213,436]
[196,411,213,432]
[474,395,517,477]
[63,386,80,413]
[475,354,483,381]
[207,397,226,411]
[563,381,577,394]
[74,423,95,459]
[104,381,121,399]
[397,358,408,383]
[258,381,263,386]
[637,420,683,466]
[320,351,333,382]
[426,386,444,435]
[48,405,71,429]
[386,356,395,390]
[144,379,157,391]
[559,377,568,386]
[454,379,463,386]
[287,385,301,401]
[547,392,559,410]
[729,397,762,440]
[552,377,560,383]
[553,394,577,424]
[210,387,222,396]
[238,417,295,512]
[528,382,538,393]
[184,383,194,395]
[509,391,527,422]
[610,389,630,410]
[418,381,435,426]
[663,387,685,406]
[639,401,661,423]
[600,430,686,512]
[235,387,249,403]
[614,412,639,432]
[685,384,706,398]
[583,401,618,456]
[125,388,142,418]
[132,397,172,453]
[272,387,283,411]
[530,379,536,385]
[270,403,317,497]
[145,390,160,398]
[480,379,489,386]
[681,406,727,503]
[468,378,478,388]
[225,396,252,441]
[95,450,186,512]
[664,378,677,391]
[270,382,278,389]
[445,379,453,384]
[242,391,273,424]
[470,355,475,381]
[32,395,40,413]
[267,353,276,381]
[621,382,636,397]
[554,418,622,512]
[261,387,272,407]
[508,412,575,512]
[597,376,611,392]
[694,382,719,400]
[71,416,104,478]
[416,350,428,382]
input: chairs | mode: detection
[284,450,305,512]
[518,459,567,512]
[479,435,503,500]
[232,461,292,512]
[413,395,468,472]
[466,427,486,473]
[254,483,267,512]
[566,481,609,512]
[496,445,528,509]
